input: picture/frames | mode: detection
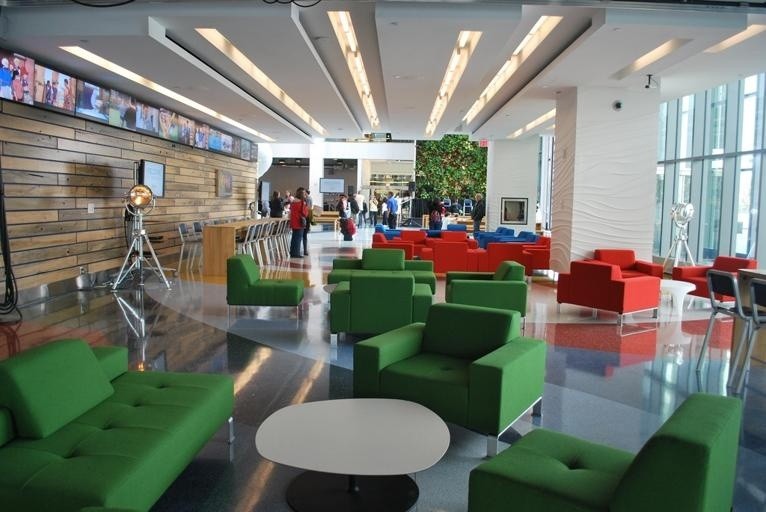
[500,196,529,226]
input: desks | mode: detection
[657,279,696,308]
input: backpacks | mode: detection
[429,211,440,222]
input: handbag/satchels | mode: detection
[346,218,356,235]
[299,217,306,227]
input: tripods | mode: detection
[110,216,177,290]
[663,229,697,268]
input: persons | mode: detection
[426,198,444,230]
[470,193,485,234]
[269,190,284,219]
[0,56,149,132]
[336,189,399,241]
[282,186,318,259]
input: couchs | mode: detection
[673,256,757,305]
[1,338,241,511]
[372,223,552,276]
[585,249,664,282]
[353,303,546,459]
[556,260,661,325]
[468,391,744,512]
[326,247,528,341]
[225,253,307,323]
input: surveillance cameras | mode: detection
[613,101,623,110]
[645,82,657,88]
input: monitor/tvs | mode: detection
[138,158,166,197]
[320,177,345,194]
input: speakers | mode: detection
[347,186,353,195]
[409,181,415,191]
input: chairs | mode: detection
[310,210,345,232]
[175,214,296,275]
[442,198,451,216]
[695,269,766,395]
[462,199,473,216]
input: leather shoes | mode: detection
[290,255,304,258]
